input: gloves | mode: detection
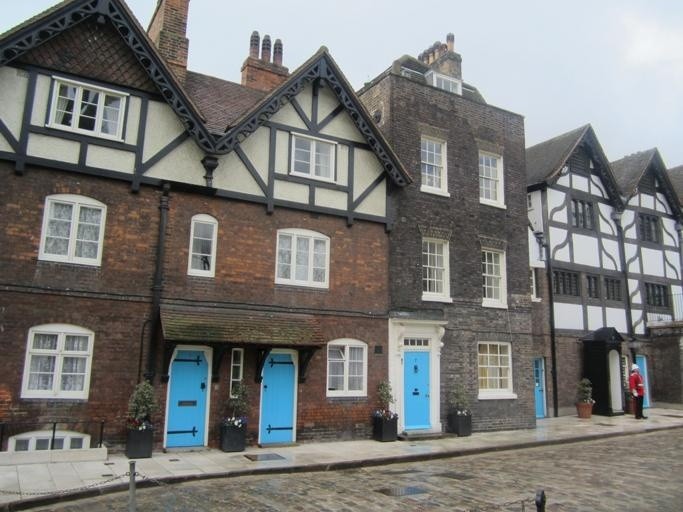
[633,390,638,397]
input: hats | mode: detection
[632,364,639,371]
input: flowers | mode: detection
[583,397,596,404]
[224,414,247,430]
[456,406,472,418]
[374,408,399,423]
[125,415,154,432]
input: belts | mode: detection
[637,384,643,387]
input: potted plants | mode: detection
[372,378,398,442]
[124,379,154,459]
[219,380,246,452]
[448,383,472,436]
[575,378,594,418]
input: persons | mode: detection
[629,363,648,420]
